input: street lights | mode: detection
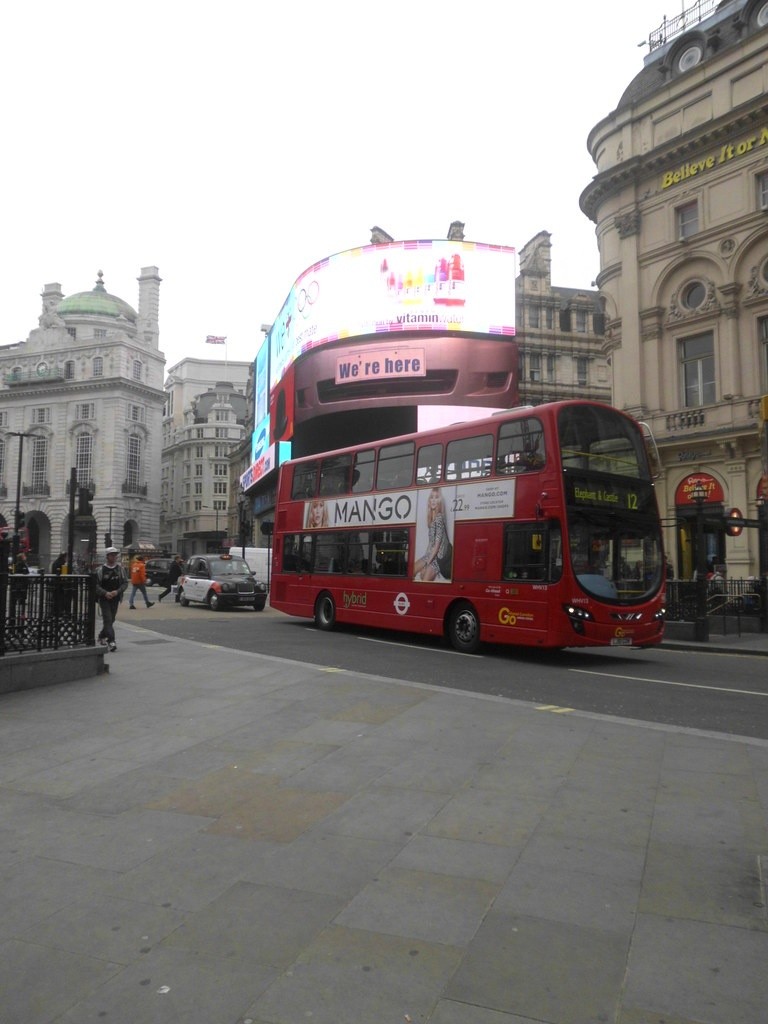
[688,478,712,643]
[202,505,219,545]
[1,431,41,567]
[105,505,118,537]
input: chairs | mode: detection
[292,477,440,499]
[286,555,407,575]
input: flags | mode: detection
[206,335,227,344]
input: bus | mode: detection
[265,398,671,656]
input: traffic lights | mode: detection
[15,511,25,528]
[103,537,112,548]
[78,487,94,516]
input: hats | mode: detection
[105,547,120,555]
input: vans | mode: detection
[228,547,272,585]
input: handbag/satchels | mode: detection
[436,544,452,579]
[711,588,723,599]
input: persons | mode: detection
[13,552,29,620]
[619,557,727,615]
[92,547,129,651]
[52,552,68,613]
[129,556,154,609]
[305,500,330,527]
[159,556,184,602]
[414,487,452,582]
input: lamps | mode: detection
[679,236,688,243]
[723,394,743,400]
[591,281,597,286]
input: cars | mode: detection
[174,553,268,612]
[144,558,175,586]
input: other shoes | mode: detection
[108,642,117,652]
[97,638,107,646]
[158,594,162,602]
[129,605,136,609]
[146,602,155,608]
